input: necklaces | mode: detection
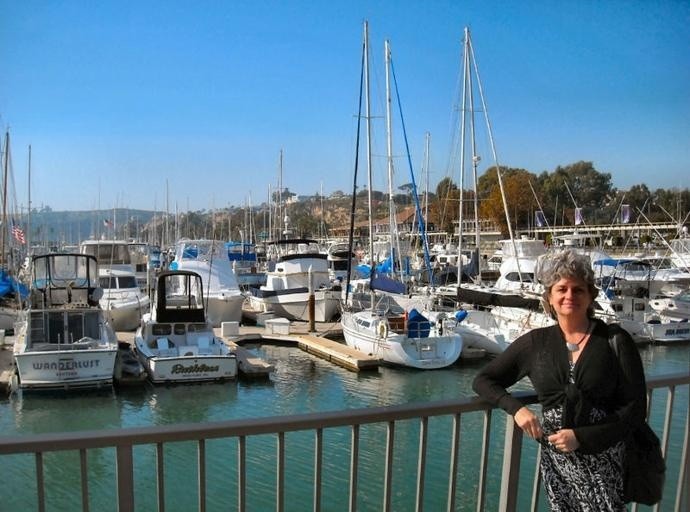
[559,318,594,352]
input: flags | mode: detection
[104,219,113,230]
[574,207,583,227]
[536,211,545,227]
[12,218,26,244]
[622,205,631,224]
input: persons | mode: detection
[471,247,648,512]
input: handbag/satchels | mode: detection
[606,323,667,508]
